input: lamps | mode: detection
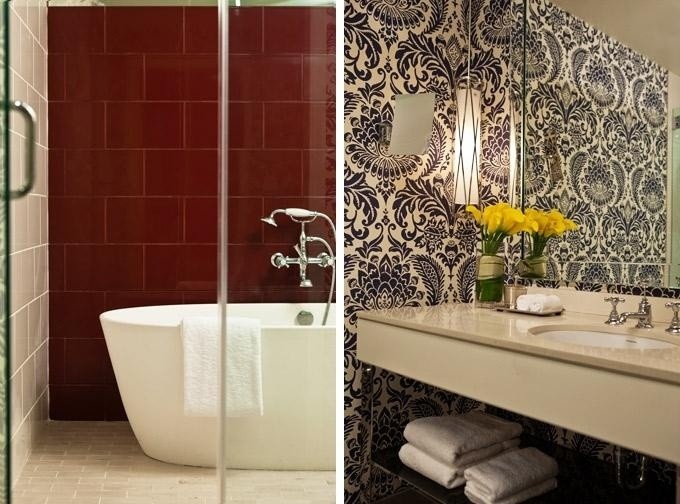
[453,78,483,205]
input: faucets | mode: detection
[619,300,654,328]
[299,277,313,288]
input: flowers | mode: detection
[466,202,537,255]
[524,205,578,255]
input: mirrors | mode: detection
[520,0,679,301]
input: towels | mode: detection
[397,407,563,501]
[178,316,269,418]
[516,292,564,314]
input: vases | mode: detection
[525,253,549,279]
[473,254,505,309]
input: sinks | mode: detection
[528,324,680,349]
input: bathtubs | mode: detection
[99,303,336,472]
